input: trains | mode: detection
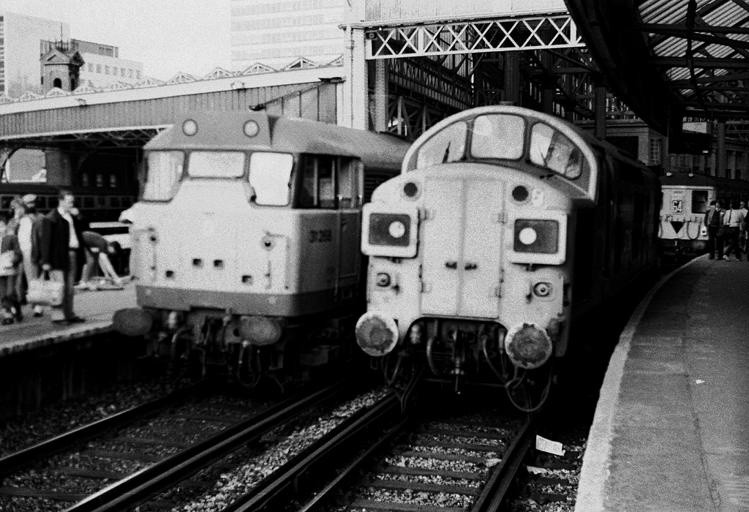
[106,108,412,406]
[354,100,749,419]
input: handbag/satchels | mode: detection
[27,270,65,306]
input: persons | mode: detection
[704,198,749,263]
[40,189,86,328]
[0,192,47,327]
[78,229,123,290]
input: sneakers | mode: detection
[710,254,742,261]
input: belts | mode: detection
[69,246,78,252]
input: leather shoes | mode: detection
[52,318,73,327]
[67,314,87,322]
[3,307,45,325]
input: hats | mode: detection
[22,194,37,203]
